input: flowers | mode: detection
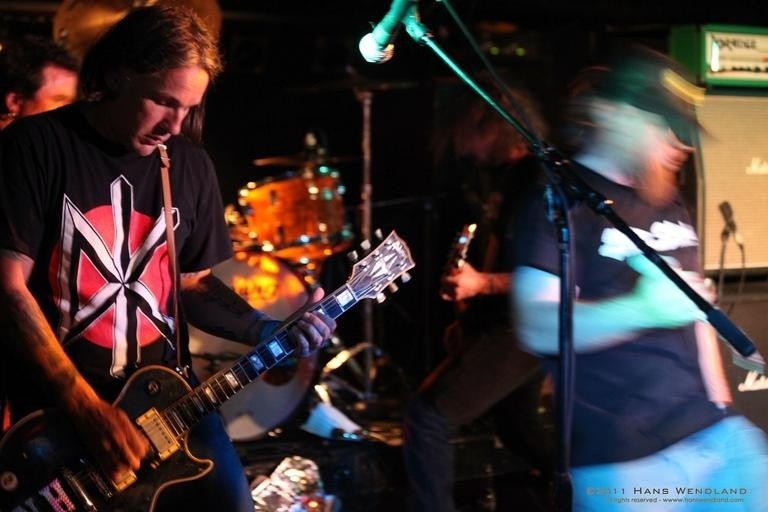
[719,200,743,246]
[357,0,415,64]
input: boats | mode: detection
[676,96,768,277]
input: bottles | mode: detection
[595,48,712,146]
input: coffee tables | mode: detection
[254,154,338,168]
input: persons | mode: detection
[1,0,768,512]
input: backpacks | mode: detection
[188,250,322,443]
[238,165,352,267]
